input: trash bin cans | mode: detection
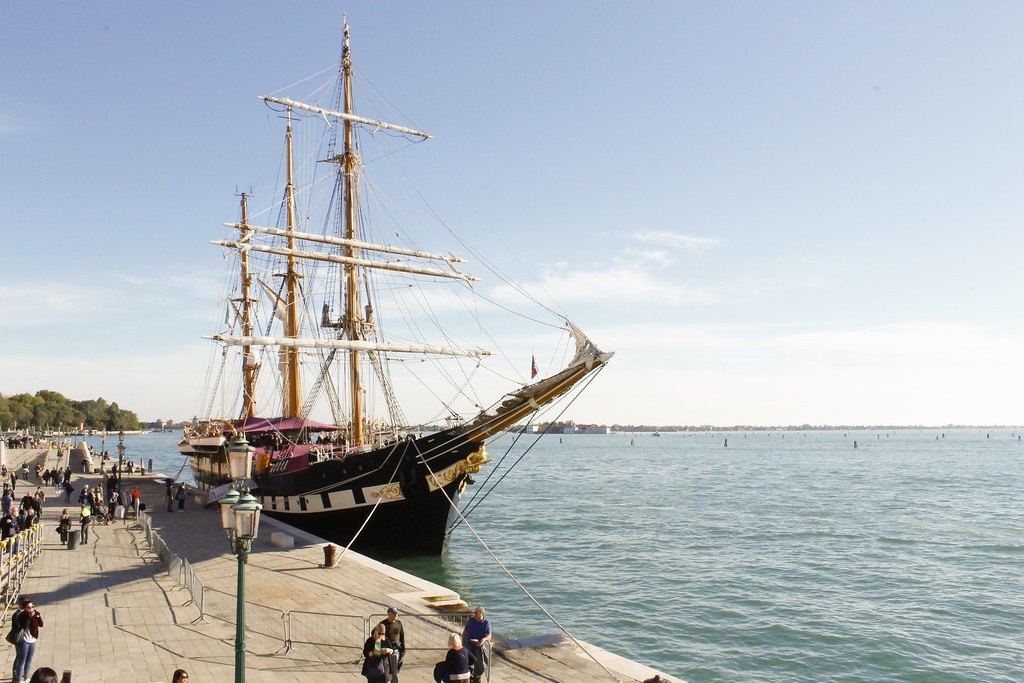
[67,530,81,550]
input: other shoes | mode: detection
[63,541,65,544]
[179,509,183,513]
[168,509,174,512]
[80,542,84,545]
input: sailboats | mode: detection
[171,7,617,557]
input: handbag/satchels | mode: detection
[5,611,24,645]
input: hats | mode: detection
[387,607,399,614]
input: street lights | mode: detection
[100,426,106,462]
[215,431,264,683]
[116,430,127,502]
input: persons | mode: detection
[127,459,134,476]
[112,464,118,475]
[316,436,330,445]
[81,457,89,473]
[79,504,91,545]
[372,608,405,683]
[11,597,43,683]
[58,509,71,545]
[446,633,477,683]
[81,476,118,525]
[132,486,139,504]
[0,460,46,554]
[105,449,110,460]
[361,623,394,683]
[8,434,40,449]
[29,667,57,683]
[43,466,75,504]
[172,669,188,683]
[462,606,492,683]
[167,484,175,512]
[175,482,187,512]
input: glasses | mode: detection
[376,631,385,636]
